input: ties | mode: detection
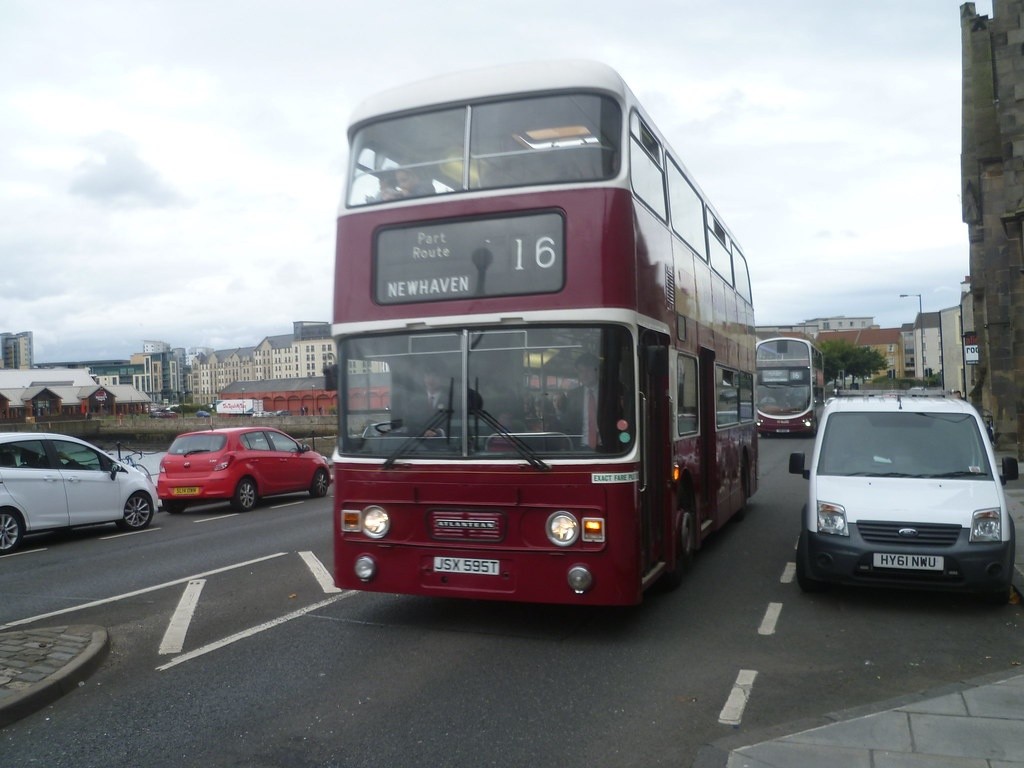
[588,390,598,450]
[429,395,439,414]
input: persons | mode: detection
[402,366,462,437]
[561,353,623,454]
[547,392,569,433]
[301,404,325,416]
[394,168,437,200]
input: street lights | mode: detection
[900,291,927,393]
[312,385,315,416]
[182,390,186,420]
[242,387,245,414]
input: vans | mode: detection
[787,389,1017,610]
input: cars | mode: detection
[148,407,288,421]
[0,434,153,550]
[156,427,335,514]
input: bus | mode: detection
[327,58,761,609]
[755,337,824,437]
